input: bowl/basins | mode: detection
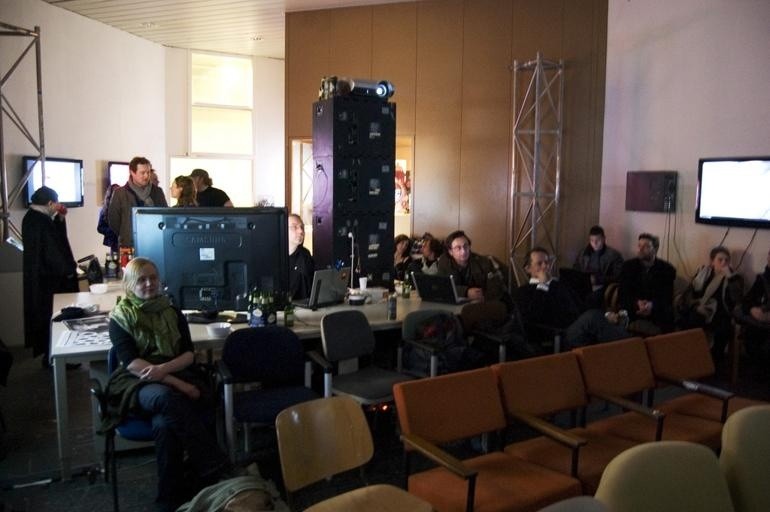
[89,283,108,294]
[206,322,232,338]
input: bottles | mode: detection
[248,288,295,327]
[105,252,119,278]
[386,278,397,320]
[403,270,411,297]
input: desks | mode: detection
[47,281,477,482]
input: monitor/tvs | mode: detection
[108,162,132,187]
[695,156,770,228]
[130,207,289,324]
[21,156,85,208]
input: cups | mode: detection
[359,278,367,289]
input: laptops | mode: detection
[411,271,475,306]
[559,269,604,293]
[292,267,351,308]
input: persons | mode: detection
[677,246,769,372]
[515,245,648,352]
[385,231,493,299]
[96,156,316,301]
[96,256,235,511]
[22,184,82,374]
[575,225,675,323]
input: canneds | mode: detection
[388,310,396,322]
[387,295,397,308]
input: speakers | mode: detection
[625,170,679,215]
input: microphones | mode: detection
[338,227,355,289]
